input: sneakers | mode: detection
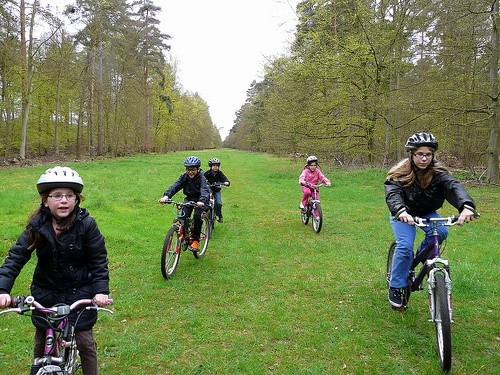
[388,287,402,308]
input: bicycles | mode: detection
[300,182,331,233]
[0,294,114,375]
[206,181,226,239]
[384,211,482,372]
[159,198,216,278]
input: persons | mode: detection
[299,156,331,223]
[384,132,478,310]
[0,166,110,375]
[158,157,211,254]
[203,157,230,225]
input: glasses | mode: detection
[413,152,433,158]
[48,194,76,202]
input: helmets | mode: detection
[306,156,318,163]
[404,133,439,153]
[208,157,221,167]
[183,156,201,167]
[36,166,85,199]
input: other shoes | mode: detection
[300,202,304,209]
[190,241,199,249]
[219,216,224,223]
